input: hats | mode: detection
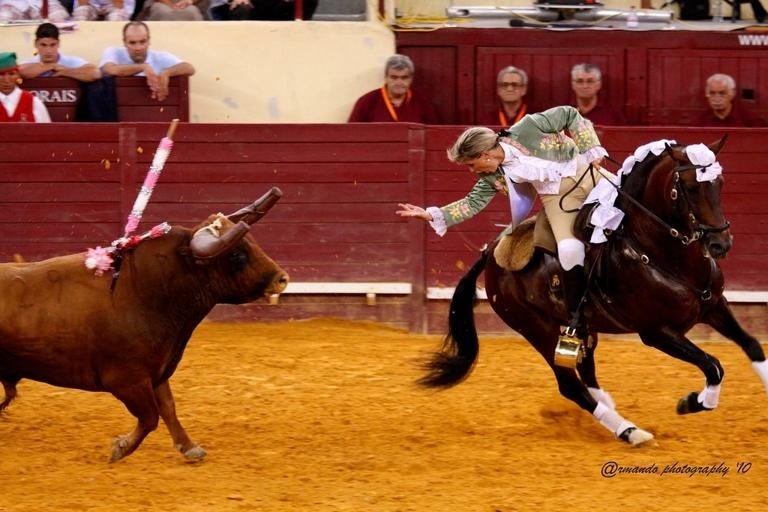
[0,51,19,76]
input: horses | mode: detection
[405,132,768,450]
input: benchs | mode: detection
[18,76,189,123]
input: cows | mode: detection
[0,185,292,467]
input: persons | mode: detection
[0,51,53,123]
[567,65,619,125]
[696,75,753,128]
[16,22,102,83]
[98,22,195,102]
[396,104,621,344]
[490,66,530,124]
[346,54,438,123]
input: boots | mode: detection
[557,260,590,344]
